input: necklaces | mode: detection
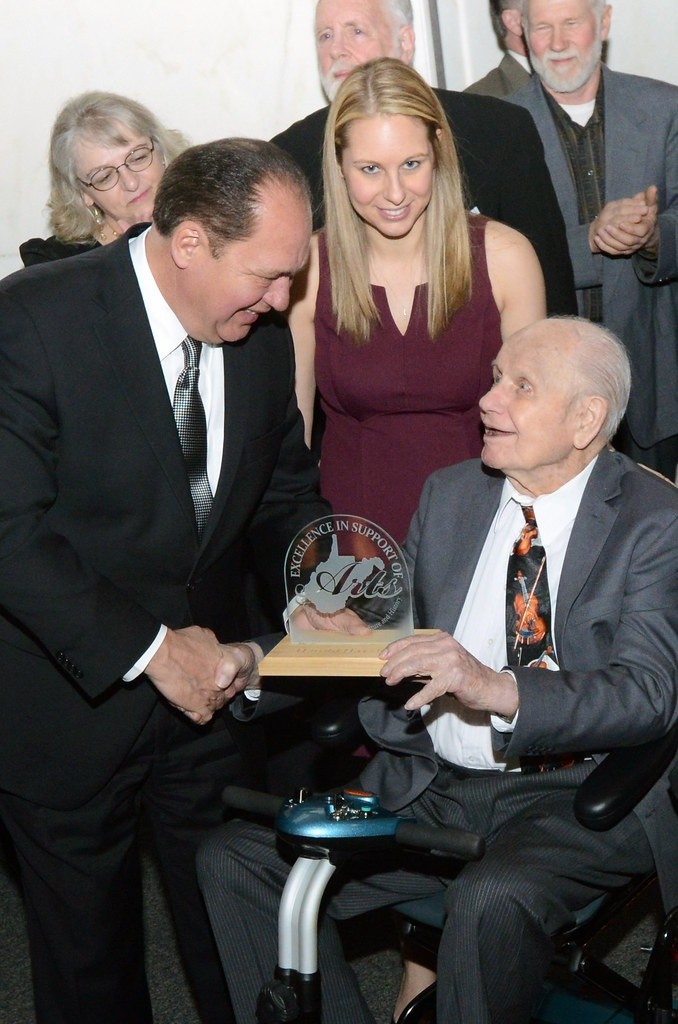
[104,218,121,237]
[384,279,421,316]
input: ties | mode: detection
[504,505,571,771]
[172,336,212,532]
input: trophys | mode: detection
[257,514,417,675]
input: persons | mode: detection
[516,2,678,477]
[203,317,678,1024]
[272,0,579,327]
[285,58,548,748]
[459,1,533,103]
[0,136,330,1024]
[22,96,204,265]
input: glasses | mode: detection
[75,138,155,192]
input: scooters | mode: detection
[258,678,678,1024]
